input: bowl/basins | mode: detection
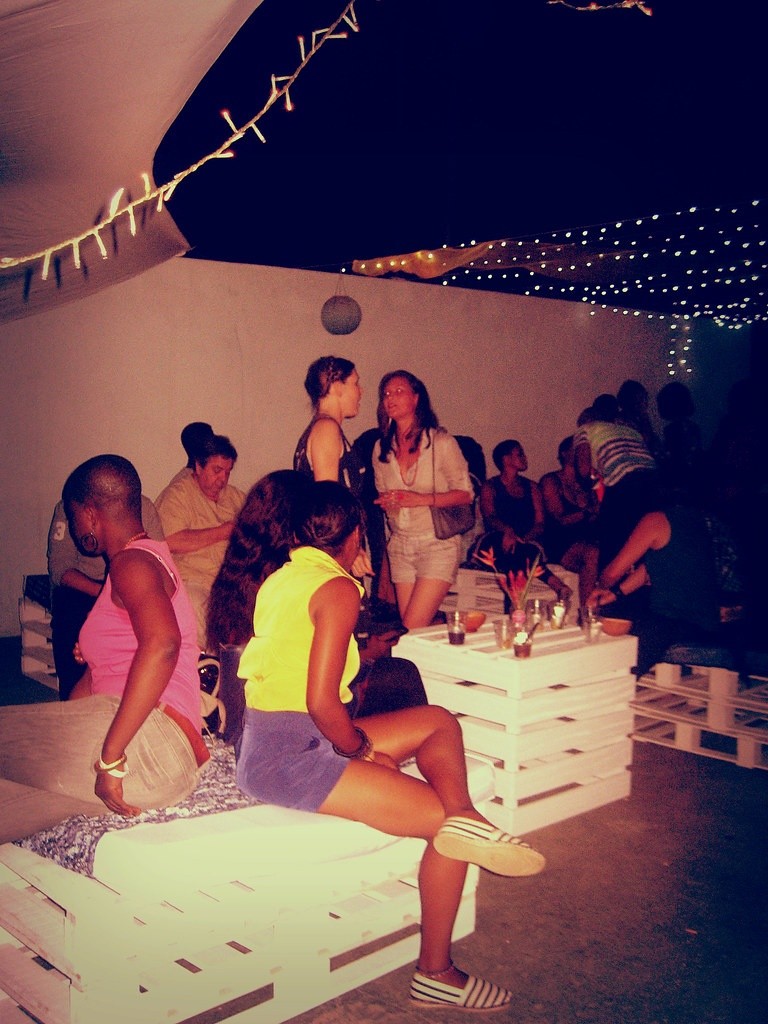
[603,617,630,636]
[465,610,485,632]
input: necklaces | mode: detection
[399,438,421,486]
[125,531,148,546]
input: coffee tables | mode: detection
[392,615,639,836]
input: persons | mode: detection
[479,379,747,681]
[47,356,475,743]
[0,454,211,845]
[237,480,545,1013]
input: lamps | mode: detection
[321,273,362,335]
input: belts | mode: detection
[155,701,210,767]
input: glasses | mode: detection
[384,389,403,398]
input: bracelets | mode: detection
[609,583,626,599]
[332,726,375,763]
[94,752,129,778]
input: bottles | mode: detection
[553,600,566,627]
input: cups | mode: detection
[584,621,604,643]
[492,618,514,649]
[512,643,531,658]
[528,599,544,632]
[446,610,466,644]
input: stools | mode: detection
[0,749,495,1024]
[18,575,59,690]
[629,642,768,772]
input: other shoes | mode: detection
[433,814,544,877]
[409,972,513,1012]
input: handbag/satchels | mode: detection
[429,504,475,539]
